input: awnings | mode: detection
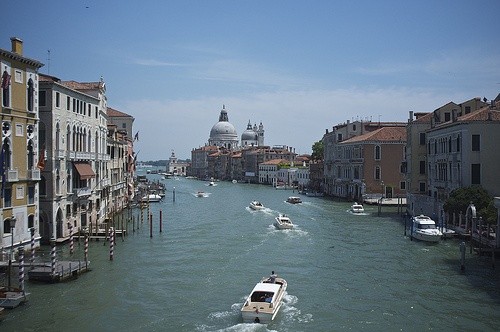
[73,161,95,180]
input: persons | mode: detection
[268,271,277,284]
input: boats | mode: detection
[407,214,444,243]
[249,202,265,210]
[241,278,288,323]
[197,191,205,197]
[210,176,215,185]
[436,226,457,239]
[350,201,365,214]
[286,196,302,204]
[137,178,166,202]
[275,213,293,230]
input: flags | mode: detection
[37,146,45,169]
